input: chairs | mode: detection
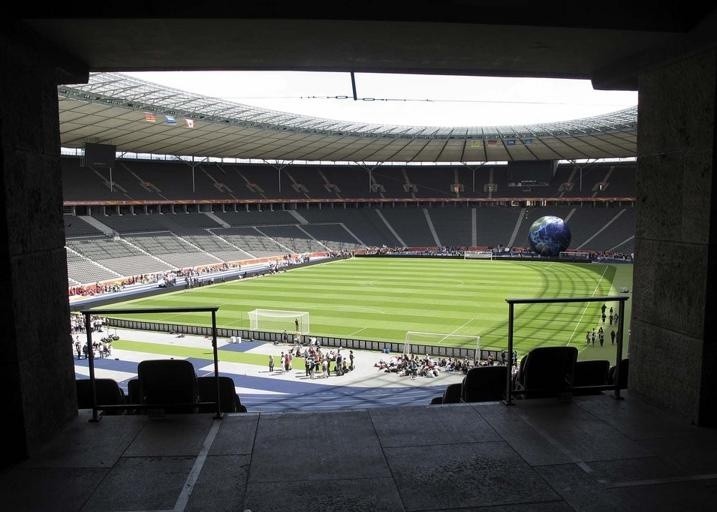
[336,161,636,264]
[429,343,628,404]
[74,355,248,415]
[61,155,230,294]
[230,163,336,281]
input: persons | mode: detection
[70,312,113,359]
[586,304,621,346]
[374,350,518,387]
[269,318,355,379]
[68,247,355,296]
[362,242,634,264]
